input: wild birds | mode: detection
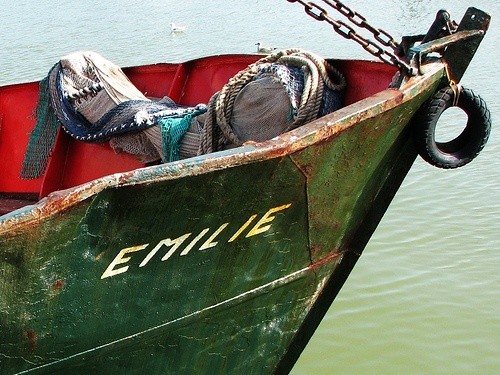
[169,23,188,32]
[255,43,277,53]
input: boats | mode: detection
[0,0,494,375]
